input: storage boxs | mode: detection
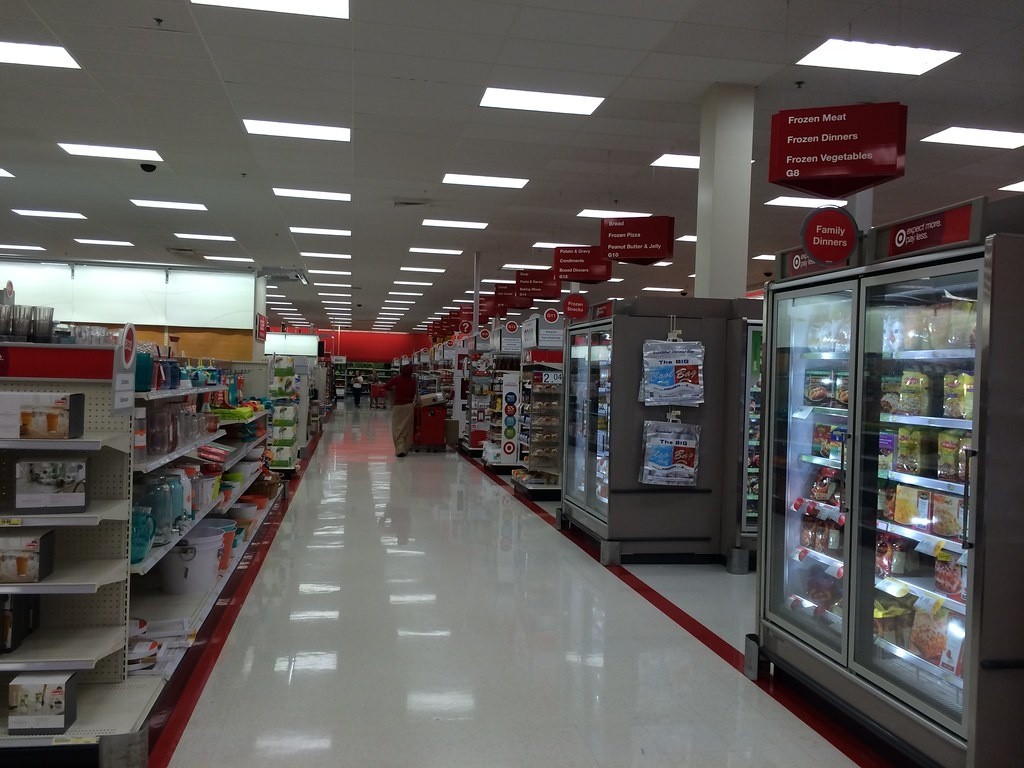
[273,375,297,395]
[273,419,297,447]
[269,445,296,469]
[275,356,295,376]
[271,405,297,426]
[420,392,446,405]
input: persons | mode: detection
[366,370,385,408]
[20,694,28,714]
[351,370,363,407]
[384,364,417,457]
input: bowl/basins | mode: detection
[227,503,259,519]
[240,496,269,510]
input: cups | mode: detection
[72,326,108,345]
[133,407,147,464]
[146,474,172,547]
[0,304,11,335]
[166,474,184,526]
[12,305,34,336]
[33,306,55,336]
[148,407,219,455]
[130,507,156,564]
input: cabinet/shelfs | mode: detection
[264,354,337,479]
[334,362,399,399]
[412,346,563,503]
[0,339,283,768]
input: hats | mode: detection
[402,364,414,376]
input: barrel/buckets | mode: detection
[159,517,237,595]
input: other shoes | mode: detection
[397,453,405,457]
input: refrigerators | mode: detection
[758,230,1024,768]
[560,315,763,542]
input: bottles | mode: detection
[185,467,199,511]
